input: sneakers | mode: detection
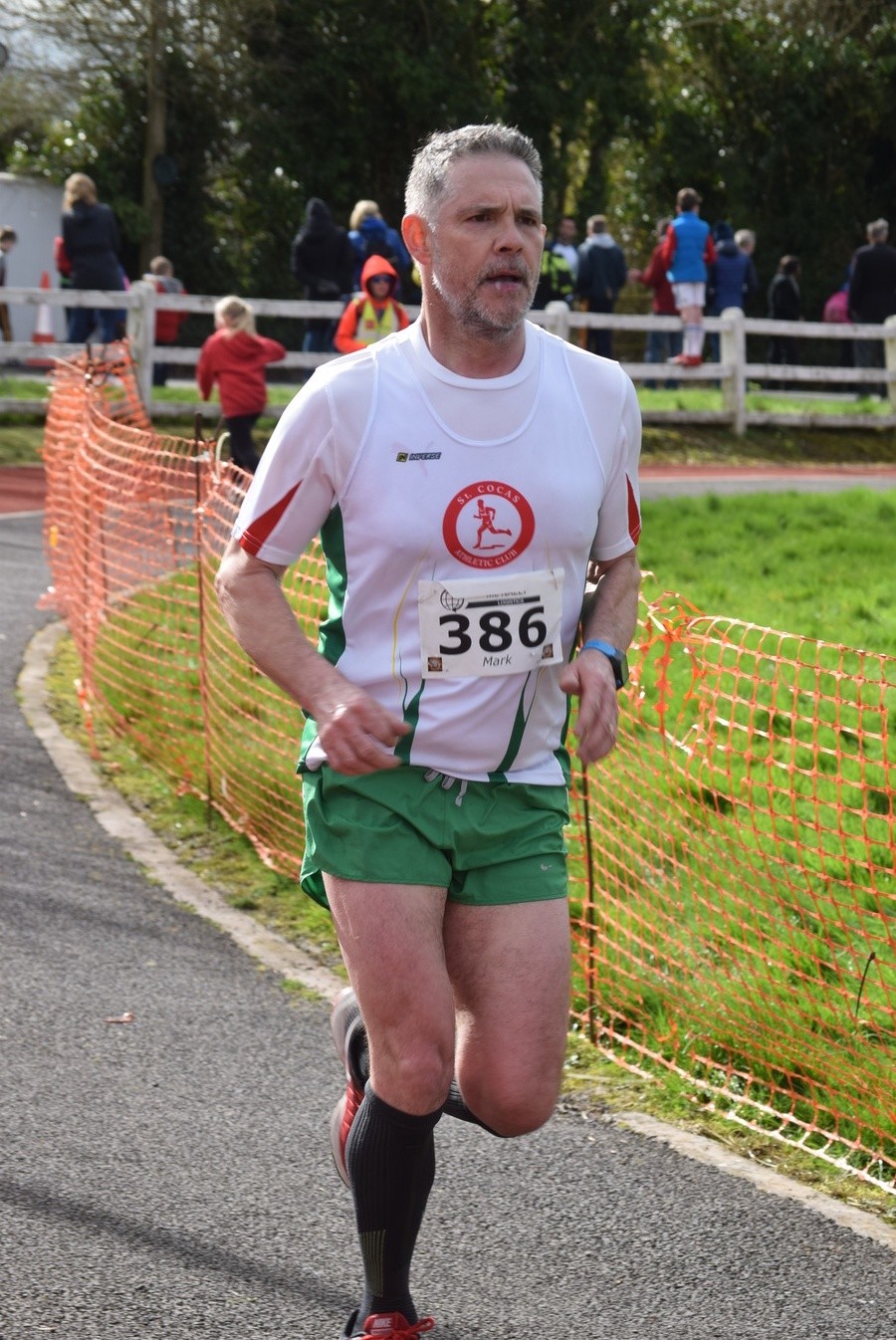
[341,1308,435,1340]
[329,985,366,1189]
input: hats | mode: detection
[677,188,702,207]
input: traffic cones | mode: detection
[29,270,59,368]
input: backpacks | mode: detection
[355,227,399,267]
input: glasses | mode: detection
[370,275,393,284]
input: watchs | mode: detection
[582,638,630,690]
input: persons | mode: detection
[197,296,287,489]
[0,224,18,341]
[217,123,642,1340]
[292,185,896,393]
[55,173,130,344]
[143,256,190,386]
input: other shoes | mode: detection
[667,353,702,367]
